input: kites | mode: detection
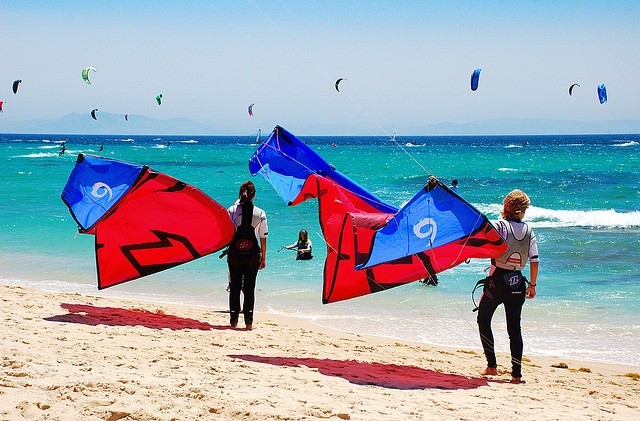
[249,125,509,303]
[61,154,237,289]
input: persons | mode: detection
[477,188,540,383]
[449,178,461,190]
[226,181,268,331]
[97,145,104,152]
[287,229,312,259]
[59,146,67,157]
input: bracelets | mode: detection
[529,283,537,287]
